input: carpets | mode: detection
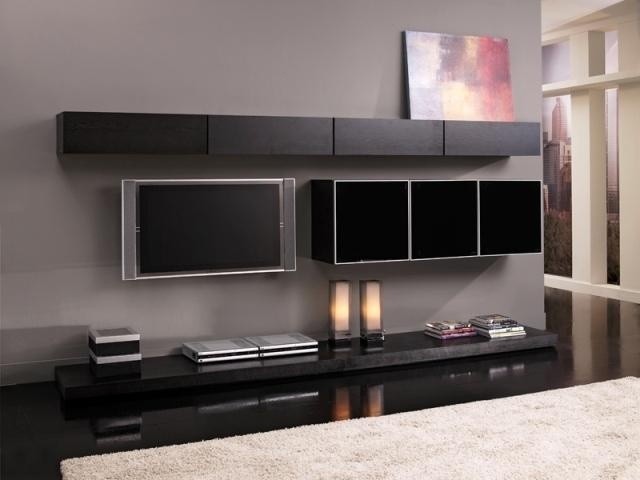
[59,374,639,480]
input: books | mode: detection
[469,314,527,339]
[424,320,477,340]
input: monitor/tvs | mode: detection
[121,176,297,285]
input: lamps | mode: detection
[329,279,353,352]
[359,279,382,345]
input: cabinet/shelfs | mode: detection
[57,109,558,404]
